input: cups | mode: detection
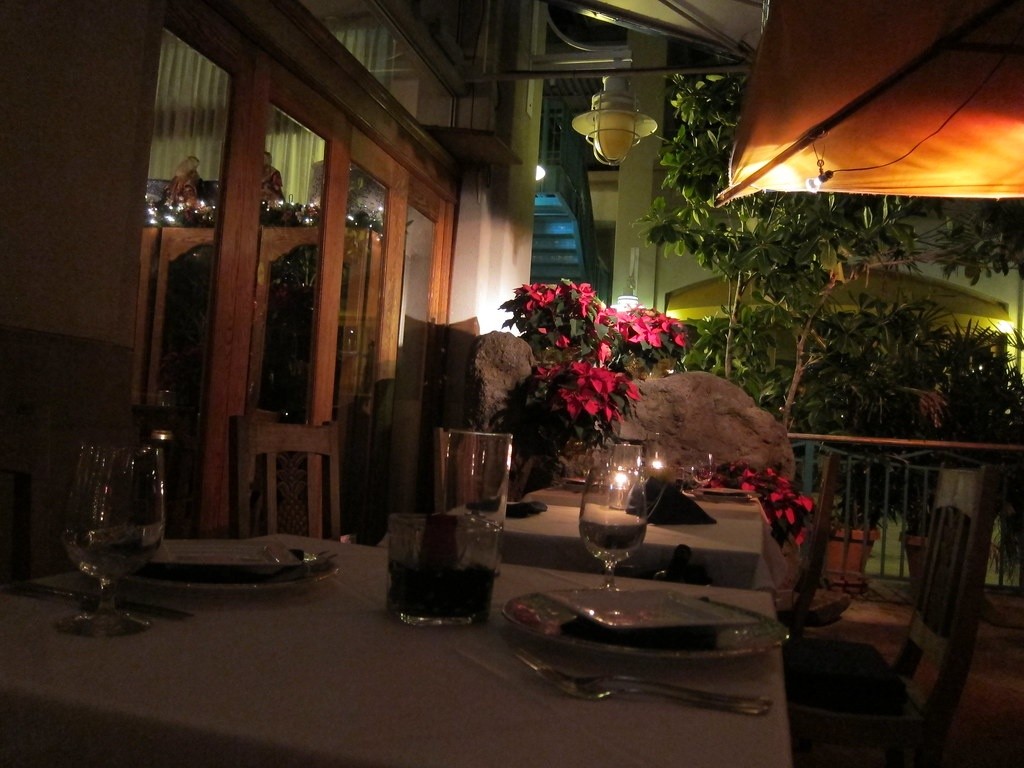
[387,513,502,627]
[435,426,513,575]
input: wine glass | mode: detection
[691,452,715,499]
[578,446,647,590]
[53,440,165,636]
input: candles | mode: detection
[607,465,644,510]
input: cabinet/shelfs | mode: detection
[146,226,383,423]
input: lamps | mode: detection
[572,76,657,166]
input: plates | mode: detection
[502,594,789,666]
[128,539,340,600]
[552,477,603,492]
[696,488,762,501]
[542,588,763,649]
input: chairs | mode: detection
[228,414,341,542]
[433,425,479,514]
[784,459,1002,768]
[773,450,850,641]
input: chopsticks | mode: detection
[9,578,195,620]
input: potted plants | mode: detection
[797,288,952,595]
[892,318,1024,591]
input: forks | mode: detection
[511,646,772,717]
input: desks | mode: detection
[0,535,794,768]
[378,487,792,592]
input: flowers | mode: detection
[523,359,641,487]
[503,278,691,369]
[695,461,815,550]
